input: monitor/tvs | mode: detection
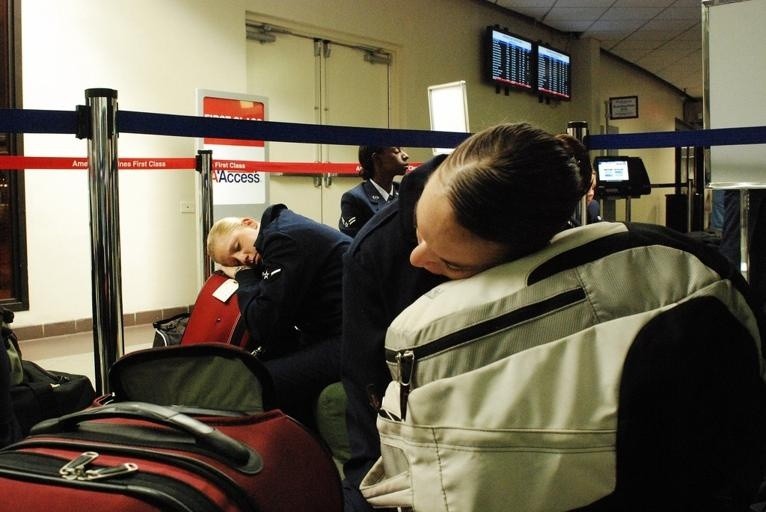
[595,156,630,184]
[535,42,571,100]
[486,25,534,91]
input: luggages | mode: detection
[0,343,345,512]
[152,272,250,350]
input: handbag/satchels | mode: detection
[359,220,766,512]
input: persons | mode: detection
[338,123,593,512]
[205,207,353,414]
[565,166,601,232]
[338,144,409,241]
